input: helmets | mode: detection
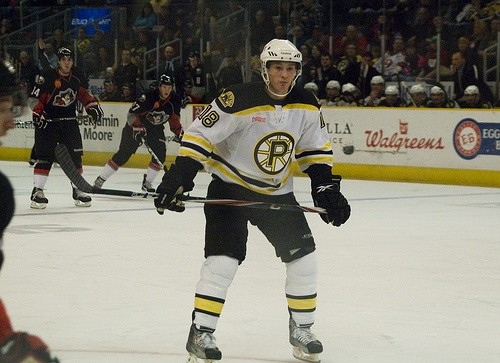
[430,87,444,95]
[0,59,19,96]
[371,76,386,85]
[411,84,425,93]
[56,47,72,57]
[327,81,340,91]
[384,86,400,95]
[160,74,173,86]
[342,84,356,94]
[304,82,318,91]
[464,85,479,96]
[259,38,303,83]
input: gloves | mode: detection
[86,106,104,123]
[154,156,204,215]
[34,111,49,132]
[310,175,350,227]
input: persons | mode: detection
[28,47,103,209]
[0,60,58,363]
[0,1,500,109]
[93,74,184,193]
[154,39,350,363]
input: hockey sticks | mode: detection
[13,115,88,124]
[55,144,327,213]
[141,137,168,173]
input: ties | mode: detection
[166,63,170,73]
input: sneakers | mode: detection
[186,310,222,363]
[73,188,92,208]
[287,305,322,363]
[142,173,155,194]
[94,176,106,188]
[30,186,48,210]
